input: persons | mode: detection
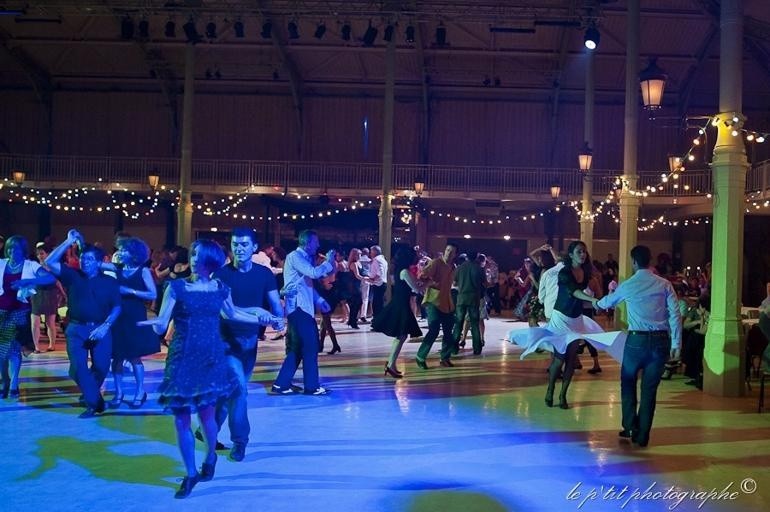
[0,227,770,500]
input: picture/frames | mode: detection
[672,293,769,415]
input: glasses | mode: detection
[411,175,426,204]
[576,139,622,211]
[145,165,162,192]
[422,65,563,92]
[549,176,584,224]
[147,59,282,84]
[578,9,605,52]
[111,7,465,52]
[662,139,685,176]
[11,166,28,192]
[636,53,719,171]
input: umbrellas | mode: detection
[269,332,341,394]
[543,361,650,448]
[0,378,21,398]
[383,338,485,379]
[174,425,247,498]
[76,393,148,418]
